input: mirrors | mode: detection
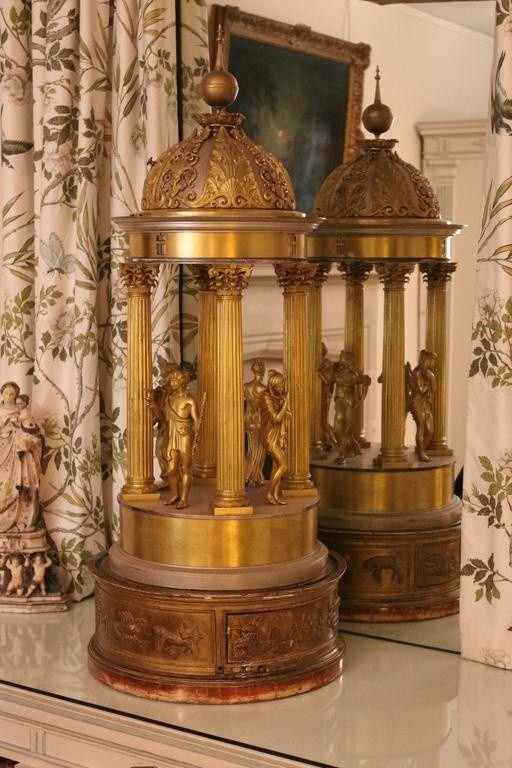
[175,0,500,660]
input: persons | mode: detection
[15,394,38,434]
[378,349,438,463]
[152,363,199,509]
[243,358,292,505]
[0,382,40,531]
[25,553,53,597]
[5,557,26,597]
[316,344,371,465]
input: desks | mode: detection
[2,585,512,767]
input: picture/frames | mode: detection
[207,4,371,223]
